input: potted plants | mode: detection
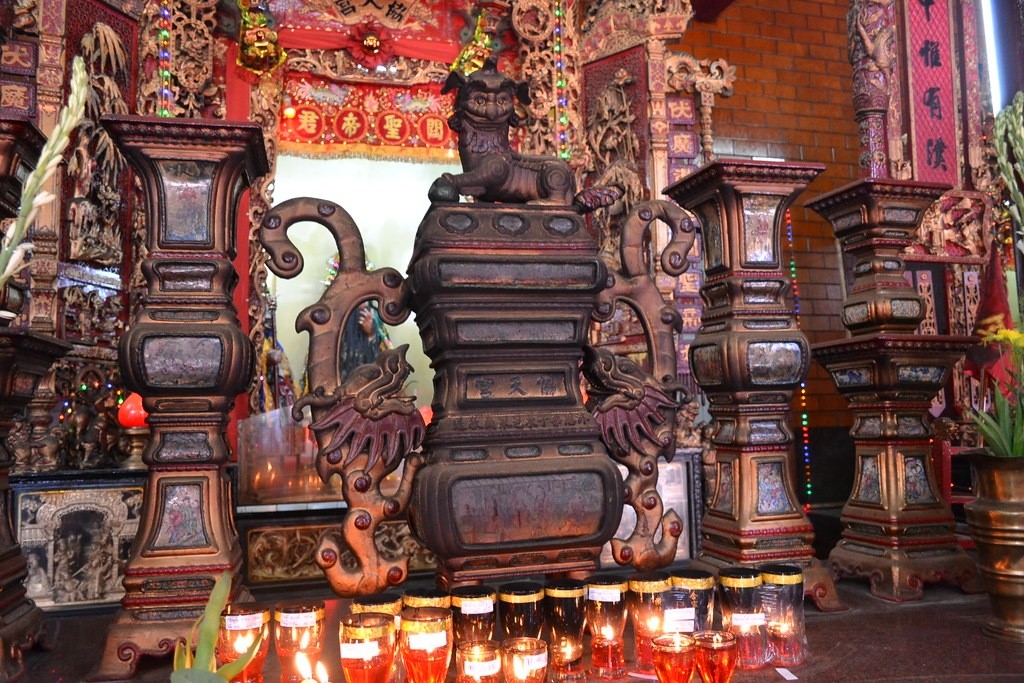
[957,356,1024,645]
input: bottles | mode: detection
[218,598,326,683]
[717,563,810,671]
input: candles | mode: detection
[220,563,811,682]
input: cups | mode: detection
[337,569,738,683]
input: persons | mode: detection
[54,532,112,602]
[301,253,394,395]
